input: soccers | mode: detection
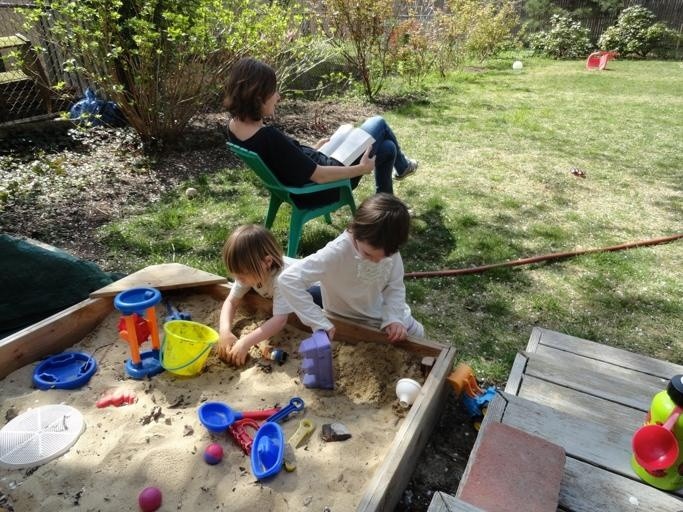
[512,60,521,68]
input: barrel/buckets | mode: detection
[159,319,221,378]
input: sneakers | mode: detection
[392,158,419,181]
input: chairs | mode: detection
[222,140,361,258]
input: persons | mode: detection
[223,58,419,211]
[217,192,424,367]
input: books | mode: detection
[316,125,376,166]
